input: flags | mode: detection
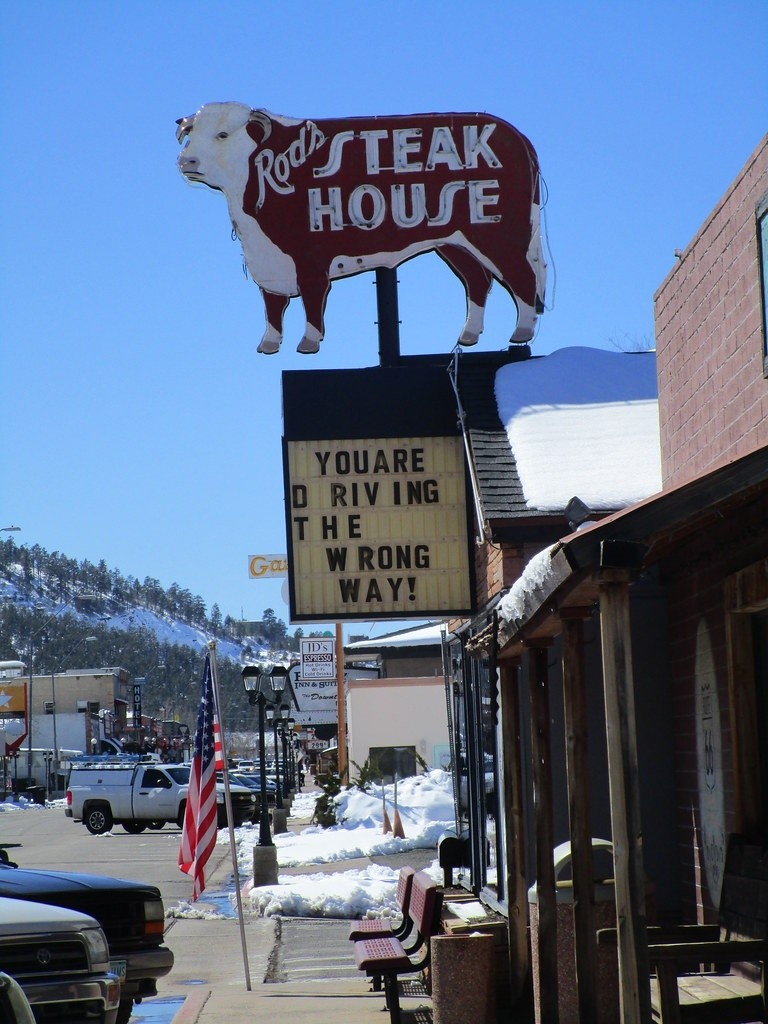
[177,654,223,902]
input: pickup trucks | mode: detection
[64,764,256,835]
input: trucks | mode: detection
[0,712,124,789]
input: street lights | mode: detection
[7,749,21,791]
[26,594,97,801]
[241,664,288,846]
[277,718,297,798]
[42,752,52,797]
[152,737,156,754]
[145,736,149,755]
[265,703,291,807]
[163,738,184,762]
[121,738,127,756]
[51,636,97,797]
[91,737,97,753]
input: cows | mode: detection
[174,101,546,356]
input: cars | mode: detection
[216,758,286,808]
[0,855,175,1024]
[0,897,120,1024]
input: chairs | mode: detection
[597,829,768,1024]
[349,864,445,1024]
